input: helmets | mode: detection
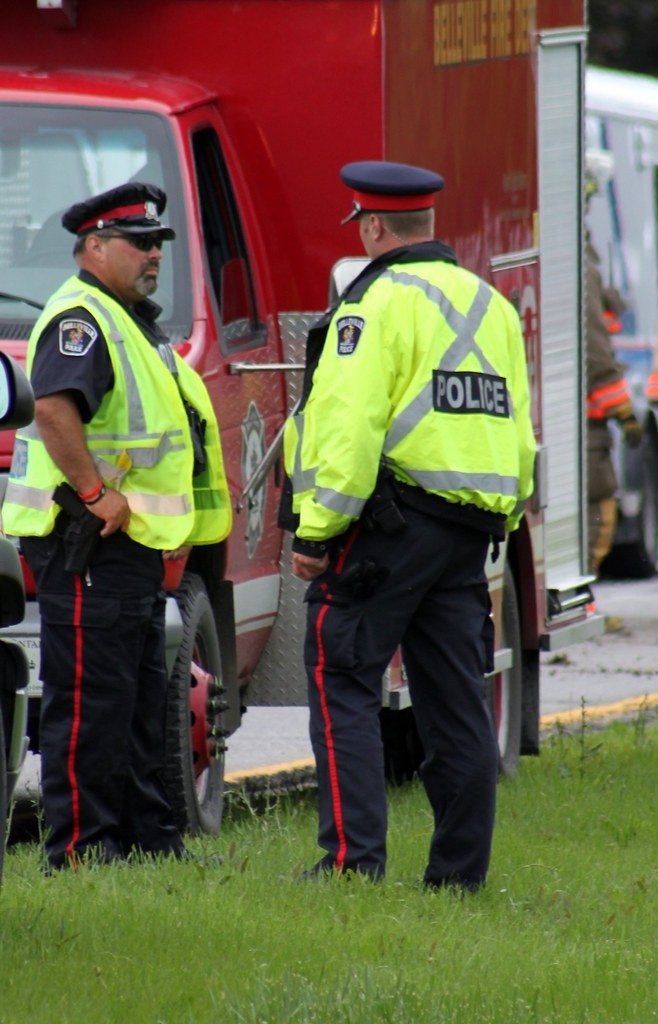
[583,168,600,209]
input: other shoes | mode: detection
[602,613,623,632]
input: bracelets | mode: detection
[83,485,106,505]
[77,481,102,498]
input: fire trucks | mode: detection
[0,0,604,843]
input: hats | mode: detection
[338,161,446,228]
[61,182,177,242]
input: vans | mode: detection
[580,62,657,575]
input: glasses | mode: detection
[96,233,165,253]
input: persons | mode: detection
[2,182,231,873]
[278,162,534,899]
[580,148,658,629]
